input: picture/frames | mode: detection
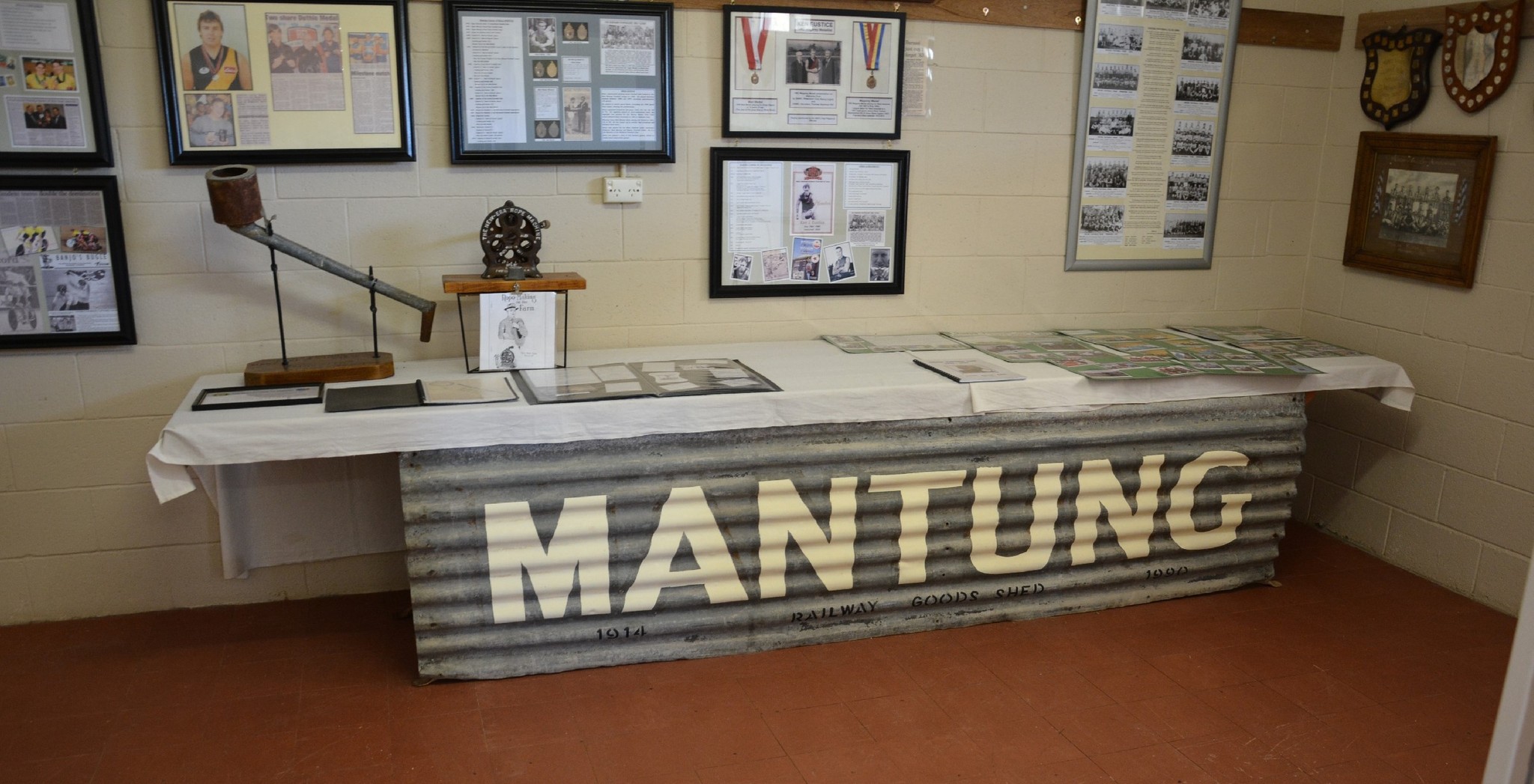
[0,0,115,169]
[1342,131,1498,288]
[0,175,137,348]
[150,0,417,165]
[721,4,907,138]
[192,381,325,411]
[443,0,678,164]
[709,147,911,299]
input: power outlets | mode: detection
[603,177,644,203]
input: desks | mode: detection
[145,338,1416,678]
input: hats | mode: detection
[504,303,518,311]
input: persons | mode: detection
[267,24,342,73]
[499,303,528,346]
[23,104,66,129]
[566,96,588,133]
[350,33,389,63]
[184,94,236,146]
[602,25,654,50]
[27,60,76,91]
[792,48,836,83]
[849,212,883,231]
[4,229,99,332]
[734,247,889,281]
[183,9,251,90]
[1083,0,1231,238]
[529,21,556,53]
[1382,182,1451,239]
[795,184,815,220]
